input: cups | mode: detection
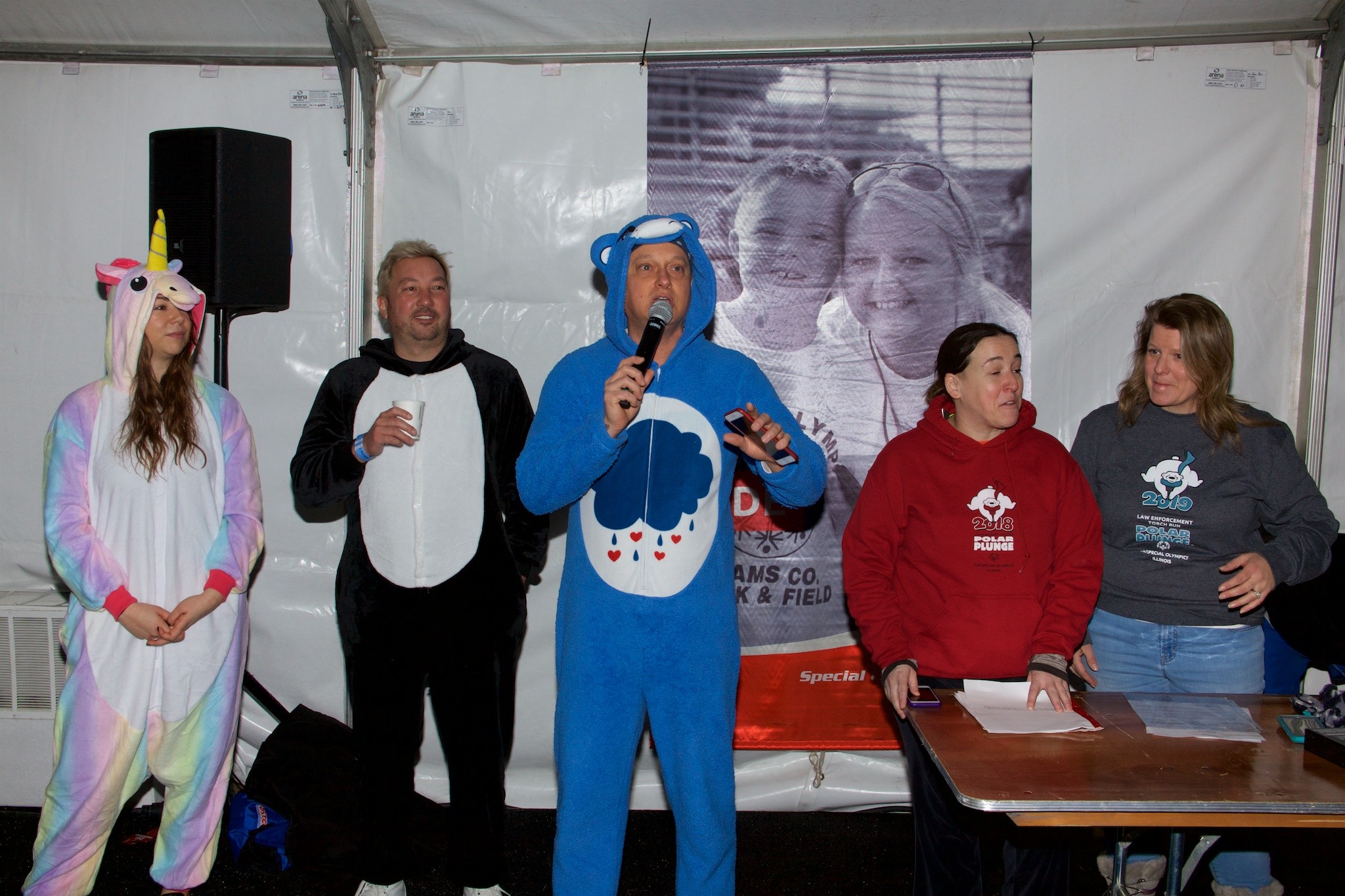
[392,398,425,442]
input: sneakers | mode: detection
[353,880,407,896]
[461,883,510,896]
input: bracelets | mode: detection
[355,433,375,462]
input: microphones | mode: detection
[618,298,673,408]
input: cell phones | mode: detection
[723,407,799,467]
[906,685,941,707]
[834,463,863,502]
[1276,712,1326,743]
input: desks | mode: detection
[897,677,1345,895]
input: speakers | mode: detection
[149,129,292,315]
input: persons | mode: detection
[704,144,887,654]
[839,321,1103,895]
[18,258,263,896]
[819,147,1031,439]
[511,214,828,896]
[287,240,550,896]
[1065,291,1341,896]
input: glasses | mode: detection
[845,162,951,198]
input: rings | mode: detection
[1251,591,1262,598]
